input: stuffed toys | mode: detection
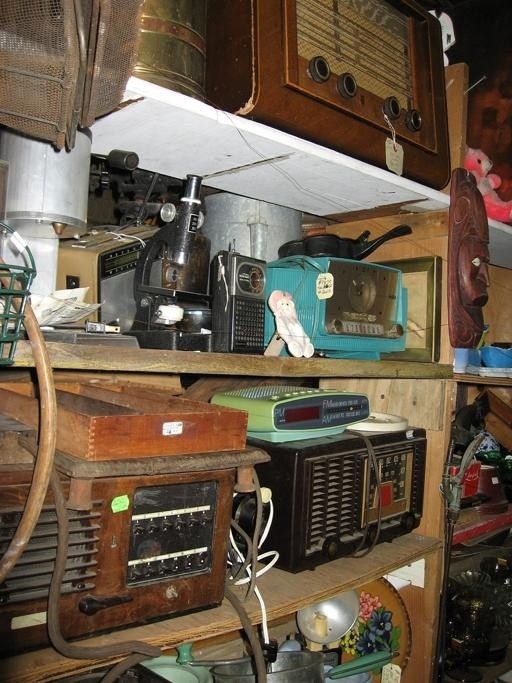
[462,144,511,222]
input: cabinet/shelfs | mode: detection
[0,28,512,681]
